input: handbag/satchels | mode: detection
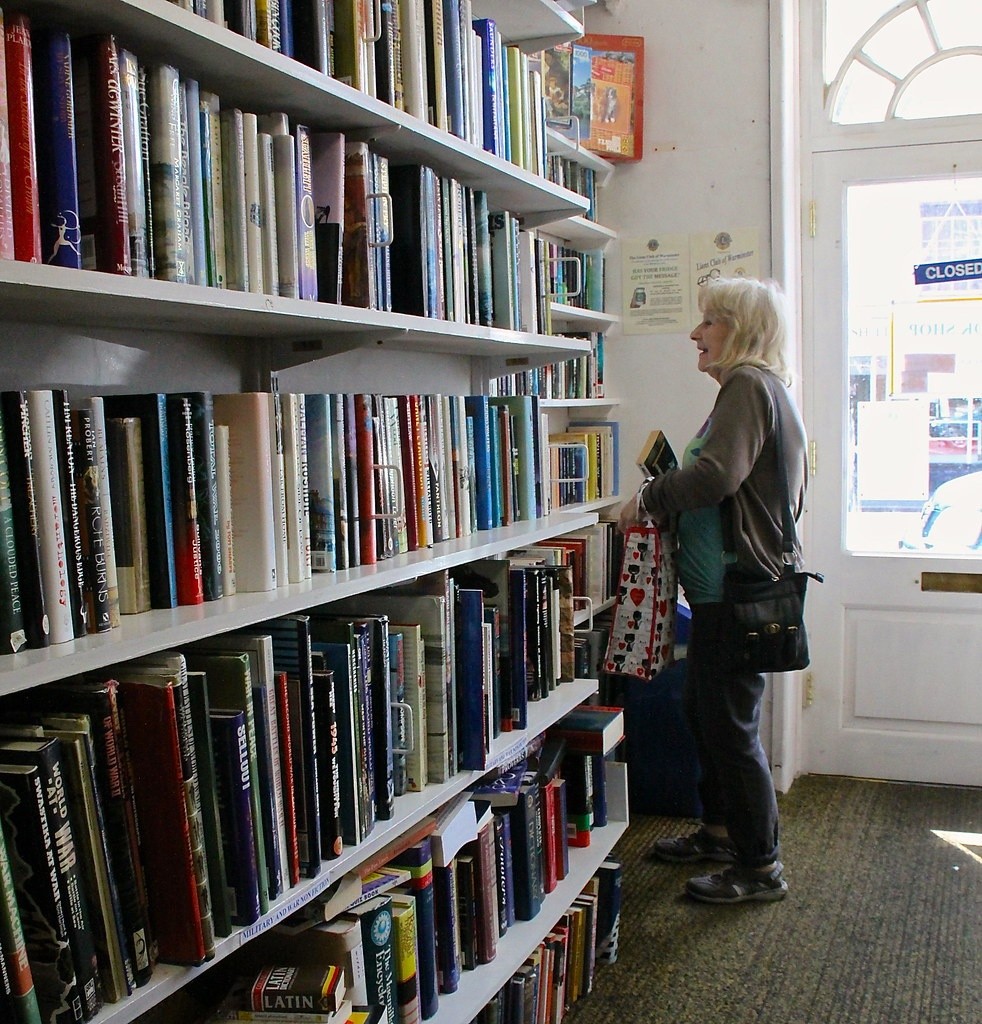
[687,561,828,673]
[602,476,679,683]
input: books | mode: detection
[0,0,645,1023]
[636,428,680,479]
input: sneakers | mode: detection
[686,864,785,903]
[653,831,737,866]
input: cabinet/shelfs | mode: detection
[0,0,634,1024]
[532,113,618,630]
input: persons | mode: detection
[616,277,809,902]
